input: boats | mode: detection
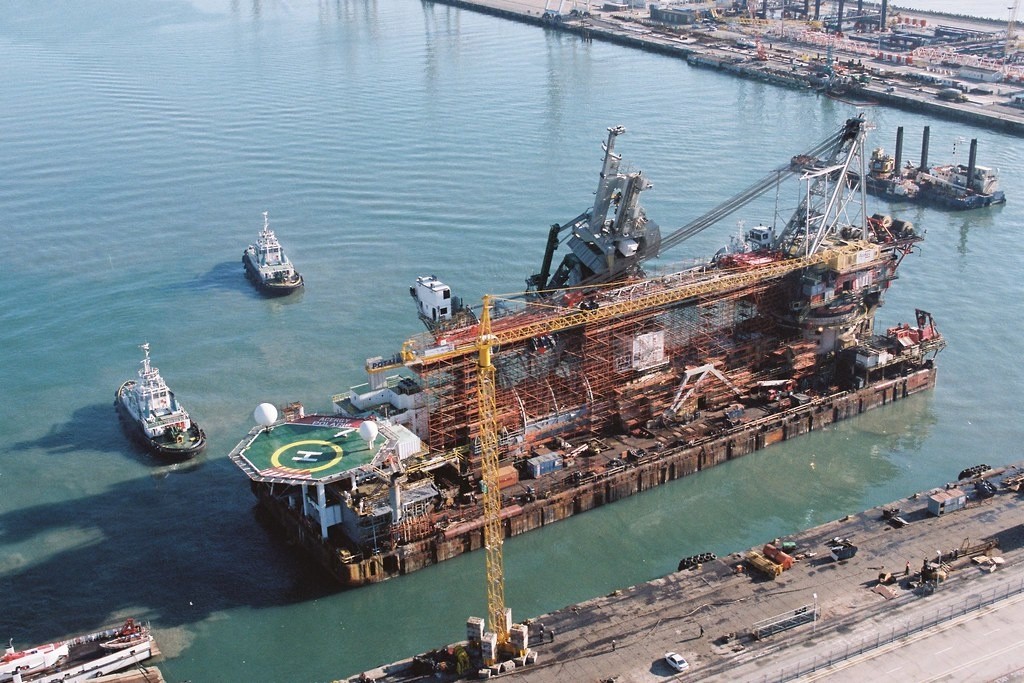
[0,619,159,683]
[114,341,207,459]
[243,210,306,296]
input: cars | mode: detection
[665,652,689,672]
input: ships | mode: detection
[790,148,921,201]
[237,108,947,589]
[902,125,1007,210]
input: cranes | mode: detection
[362,231,887,682]
